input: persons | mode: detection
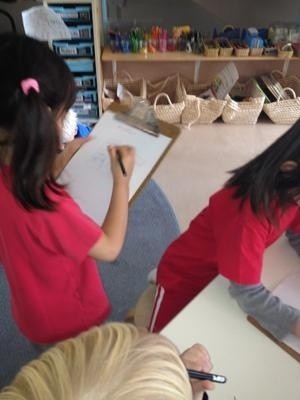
[0,37,135,362]
[0,323,216,399]
[147,117,300,344]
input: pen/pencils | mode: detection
[186,370,226,383]
[116,150,128,176]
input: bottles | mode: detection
[111,23,176,53]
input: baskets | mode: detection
[101,70,300,125]
[202,37,293,57]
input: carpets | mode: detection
[0,177,182,391]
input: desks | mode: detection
[159,232,300,400]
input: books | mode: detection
[250,70,291,102]
